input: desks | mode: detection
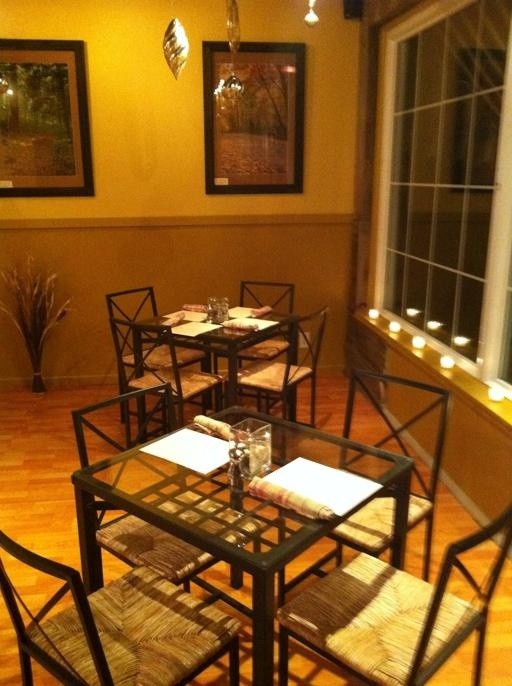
[278,369,450,610]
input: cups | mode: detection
[228,418,273,482]
[207,296,230,326]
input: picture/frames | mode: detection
[202,41,305,193]
[0,39,94,195]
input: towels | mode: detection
[182,303,209,313]
[248,475,335,520]
[193,415,249,440]
[159,311,185,332]
[223,320,258,332]
[250,306,272,317]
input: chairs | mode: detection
[0,529,242,686]
[111,319,221,462]
[273,503,511,686]
[73,383,246,617]
[235,308,329,429]
[106,286,211,422]
[214,281,295,415]
